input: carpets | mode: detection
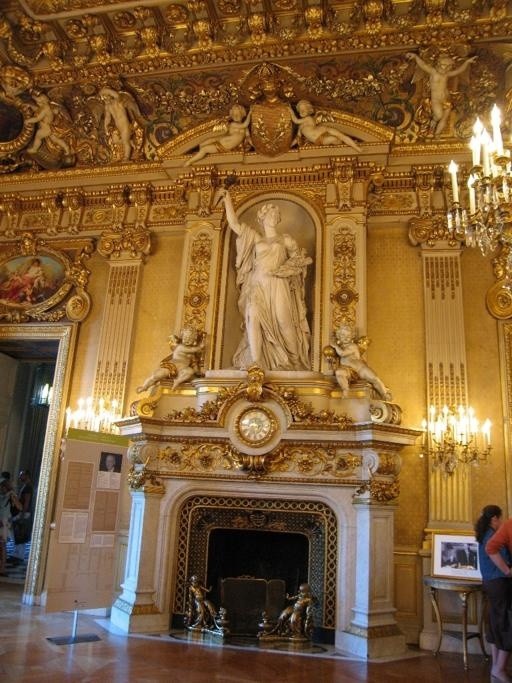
[0,535,31,583]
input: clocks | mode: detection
[235,405,277,448]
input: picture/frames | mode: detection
[429,530,484,582]
[0,246,75,312]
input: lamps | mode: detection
[446,103,511,255]
[422,406,493,476]
[60,395,122,463]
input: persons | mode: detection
[0,257,47,304]
[442,544,458,566]
[474,504,512,682]
[406,53,479,139]
[264,584,312,637]
[185,104,255,166]
[217,189,313,369]
[330,327,387,398]
[106,455,116,472]
[0,471,16,570]
[188,575,217,629]
[137,329,205,394]
[288,100,362,152]
[99,86,132,161]
[24,94,69,153]
[485,520,512,576]
[459,543,476,569]
[11,467,33,564]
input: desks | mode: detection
[425,575,489,672]
